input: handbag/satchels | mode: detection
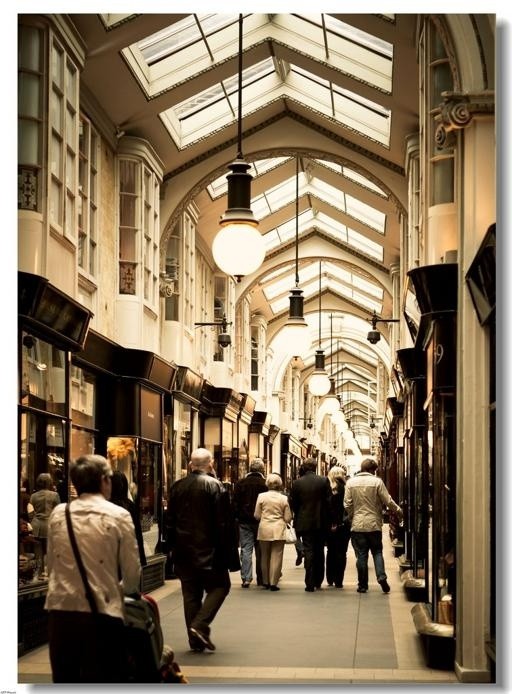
[91,614,124,676]
[284,523,298,544]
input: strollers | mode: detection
[121,593,186,684]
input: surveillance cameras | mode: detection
[367,331,381,344]
[371,452,374,456]
[307,423,313,429]
[218,334,233,348]
[344,452,346,456]
[333,445,336,448]
[370,423,375,428]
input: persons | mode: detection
[110,471,147,581]
[169,447,242,653]
[42,454,142,683]
[235,458,403,593]
[20,470,65,557]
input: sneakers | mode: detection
[190,627,215,650]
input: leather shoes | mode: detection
[296,555,303,565]
[242,580,390,595]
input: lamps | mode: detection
[194,313,234,348]
[209,14,266,282]
[304,254,365,470]
[279,149,310,359]
[369,413,384,429]
[367,308,401,344]
